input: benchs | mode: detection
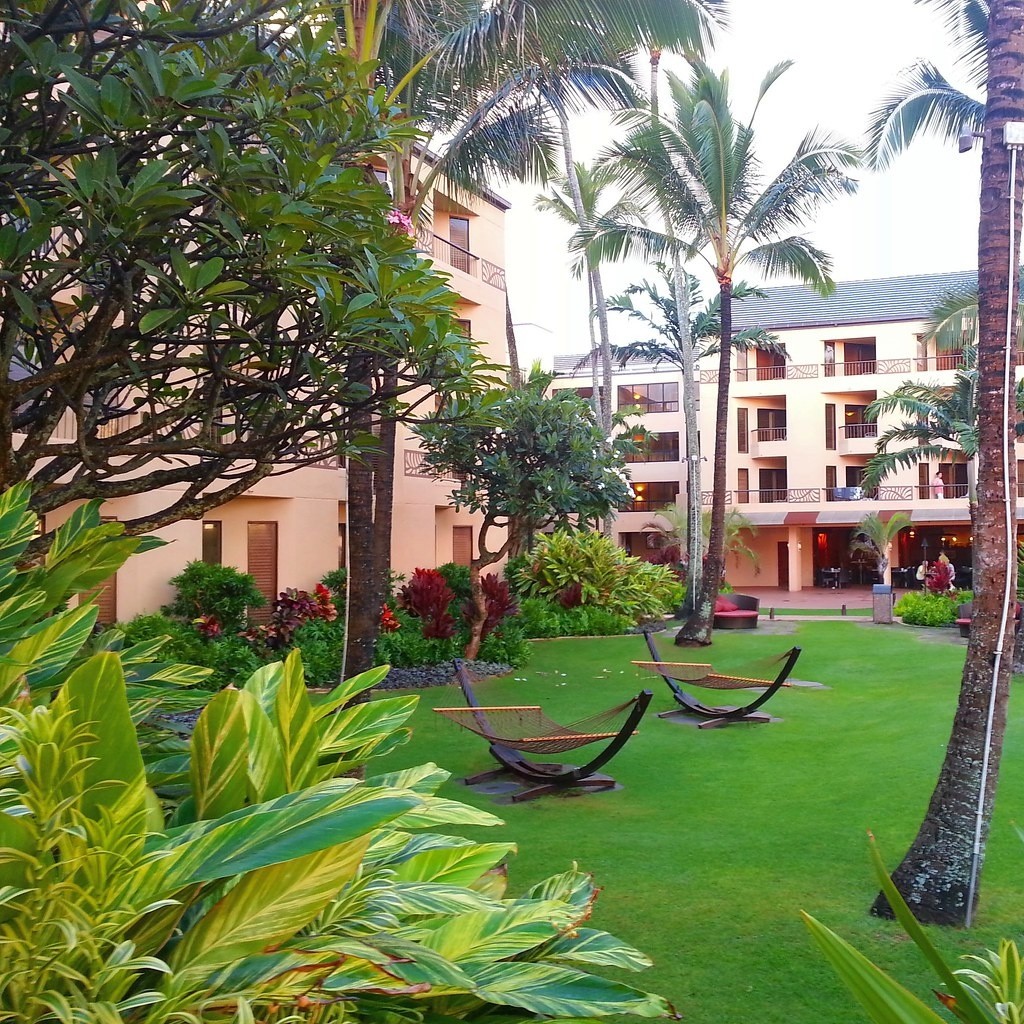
[714,593,760,630]
[954,601,1022,640]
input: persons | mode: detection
[946,560,955,590]
[916,561,928,580]
[932,471,944,499]
[939,550,949,565]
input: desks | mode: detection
[823,570,853,588]
[850,561,868,585]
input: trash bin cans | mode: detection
[871,583,893,624]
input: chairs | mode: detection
[834,569,851,589]
[911,568,925,592]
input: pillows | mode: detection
[715,595,738,613]
